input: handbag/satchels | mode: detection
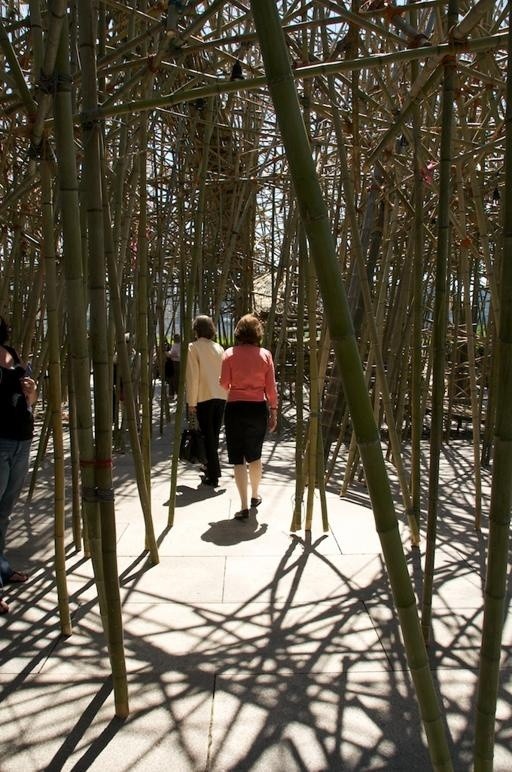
[179,413,209,465]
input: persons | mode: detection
[110,326,184,429]
[217,312,278,521]
[0,314,41,588]
[183,313,229,487]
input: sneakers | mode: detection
[1,570,29,616]
[250,494,262,507]
[236,510,250,518]
[200,469,219,487]
